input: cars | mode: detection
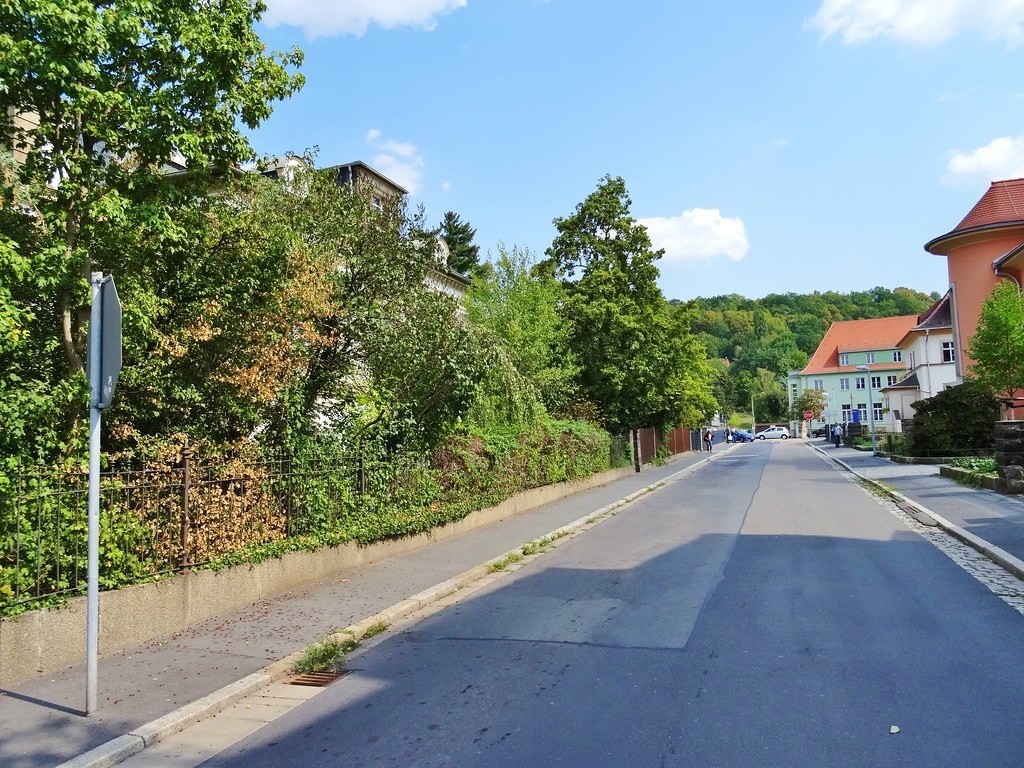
[754,427,790,440]
[807,427,826,439]
[723,428,755,443]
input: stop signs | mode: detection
[803,411,814,420]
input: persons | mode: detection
[704,430,714,453]
[725,426,730,444]
[831,422,843,448]
[731,428,737,444]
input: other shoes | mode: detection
[838,445,840,447]
[710,451,712,453]
[836,446,838,448]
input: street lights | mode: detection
[751,391,765,433]
[857,365,878,456]
